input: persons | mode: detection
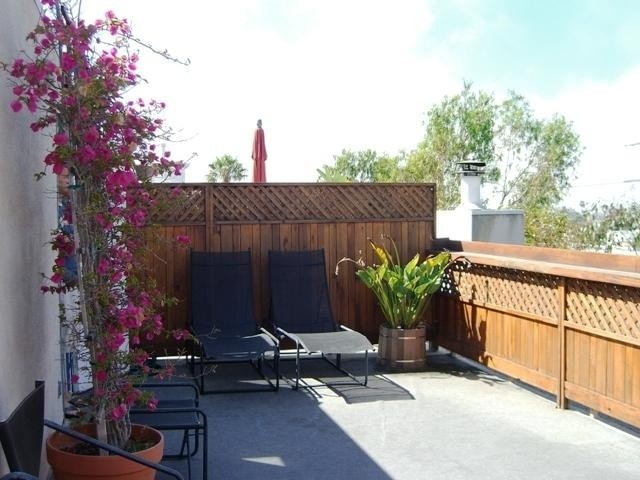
[251,119,266,184]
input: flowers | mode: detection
[0,1,197,448]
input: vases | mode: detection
[44,425,165,480]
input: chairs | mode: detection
[0,376,186,480]
[178,246,285,398]
[62,380,209,480]
[263,245,377,393]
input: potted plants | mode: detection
[328,231,473,377]
